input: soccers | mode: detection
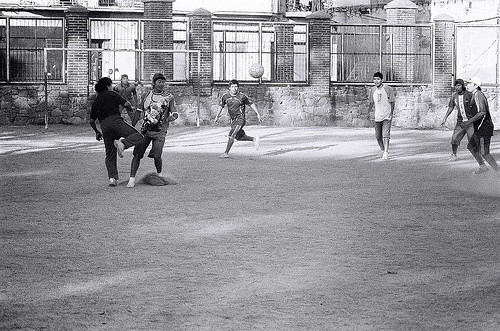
[248,63,265,78]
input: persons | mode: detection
[214,79,260,158]
[370,72,396,160]
[441,77,500,175]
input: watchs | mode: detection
[89,73,179,188]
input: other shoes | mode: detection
[109,178,119,186]
[157,168,163,177]
[220,153,229,158]
[382,152,390,160]
[114,140,124,158]
[126,177,135,188]
[472,167,489,174]
[448,156,458,162]
[255,136,260,149]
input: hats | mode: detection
[152,73,165,89]
[467,76,482,90]
